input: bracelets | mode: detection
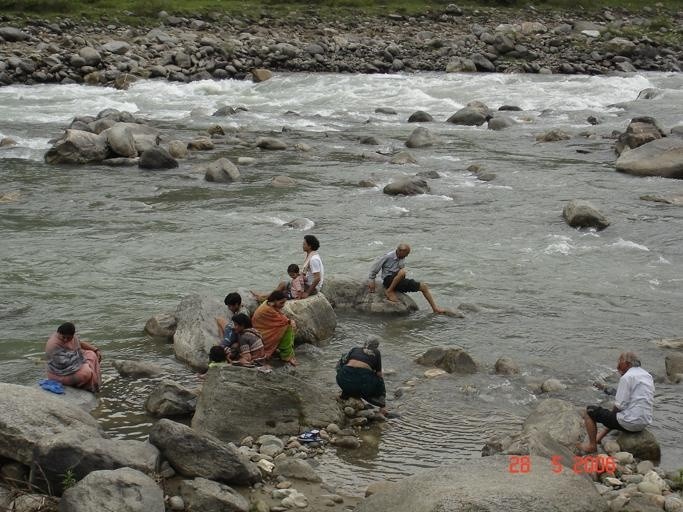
[94,348,99,352]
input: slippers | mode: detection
[39,378,65,394]
[233,360,256,368]
[298,429,323,442]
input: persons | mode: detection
[335,336,386,412]
[368,243,444,314]
[45,322,103,395]
[575,352,655,452]
[209,235,323,367]
